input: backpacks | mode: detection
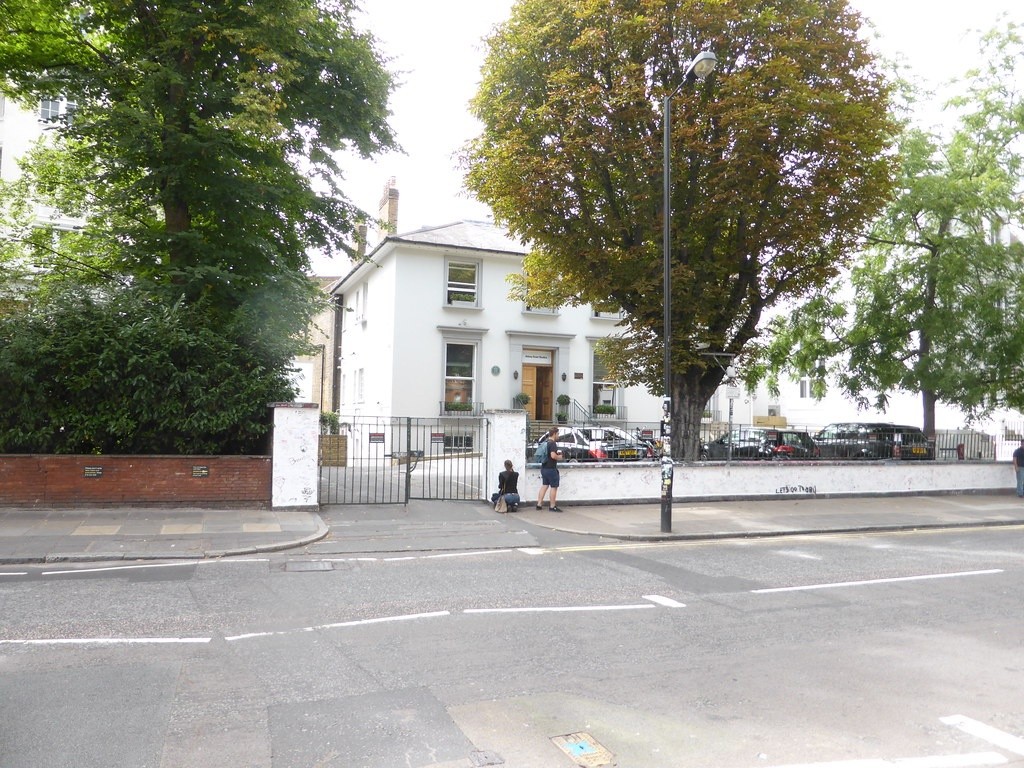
[534,441,548,463]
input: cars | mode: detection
[526,421,934,464]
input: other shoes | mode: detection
[549,506,562,512]
[536,504,542,509]
[511,504,517,511]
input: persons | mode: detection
[491,460,520,512]
[536,428,564,512]
[1013,440,1024,498]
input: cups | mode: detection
[557,450,562,455]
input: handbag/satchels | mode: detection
[495,495,507,513]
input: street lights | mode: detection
[661,51,719,529]
